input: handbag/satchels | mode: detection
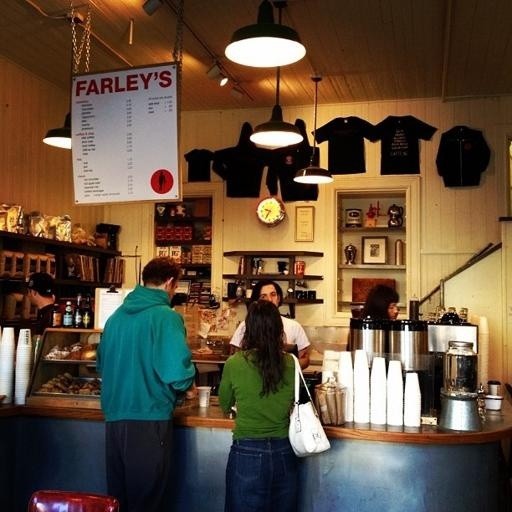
[288,402,332,458]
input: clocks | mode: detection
[256,196,285,226]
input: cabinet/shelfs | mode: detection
[223,251,324,318]
[324,176,420,327]
[141,182,224,304]
[0,230,122,335]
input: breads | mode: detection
[37,344,100,397]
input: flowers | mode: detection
[201,304,237,329]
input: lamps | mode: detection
[250,0,304,146]
[43,13,85,149]
[225,0,306,68]
[293,78,332,184]
[143,0,253,102]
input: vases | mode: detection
[207,327,233,354]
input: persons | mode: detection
[96,259,197,512]
[348,283,399,319]
[219,300,301,512]
[229,278,311,371]
[21,271,60,333]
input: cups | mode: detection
[401,370,422,429]
[0,326,15,404]
[278,260,289,273]
[15,328,33,405]
[295,260,305,275]
[196,386,214,409]
[483,395,504,411]
[353,349,370,424]
[369,354,385,426]
[336,350,353,423]
[226,283,317,300]
[488,380,501,395]
[386,359,404,427]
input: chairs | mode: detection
[28,490,119,512]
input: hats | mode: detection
[27,272,55,294]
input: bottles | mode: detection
[239,257,265,275]
[394,238,403,267]
[424,305,468,324]
[52,291,93,330]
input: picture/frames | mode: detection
[294,206,315,242]
[362,237,388,264]
